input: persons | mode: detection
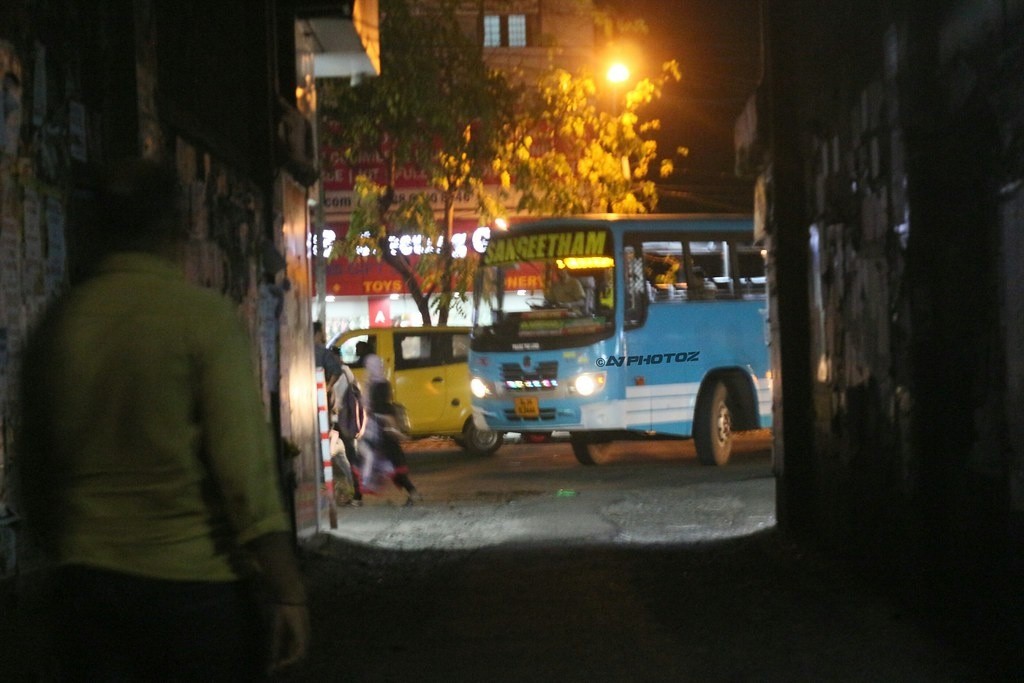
[691,269,719,300]
[543,260,585,311]
[18,152,318,682]
[311,320,423,505]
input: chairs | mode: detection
[652,277,768,300]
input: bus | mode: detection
[466,211,774,468]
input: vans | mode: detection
[323,327,504,458]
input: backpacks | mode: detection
[338,371,365,439]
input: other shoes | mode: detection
[362,488,383,501]
[402,492,419,506]
